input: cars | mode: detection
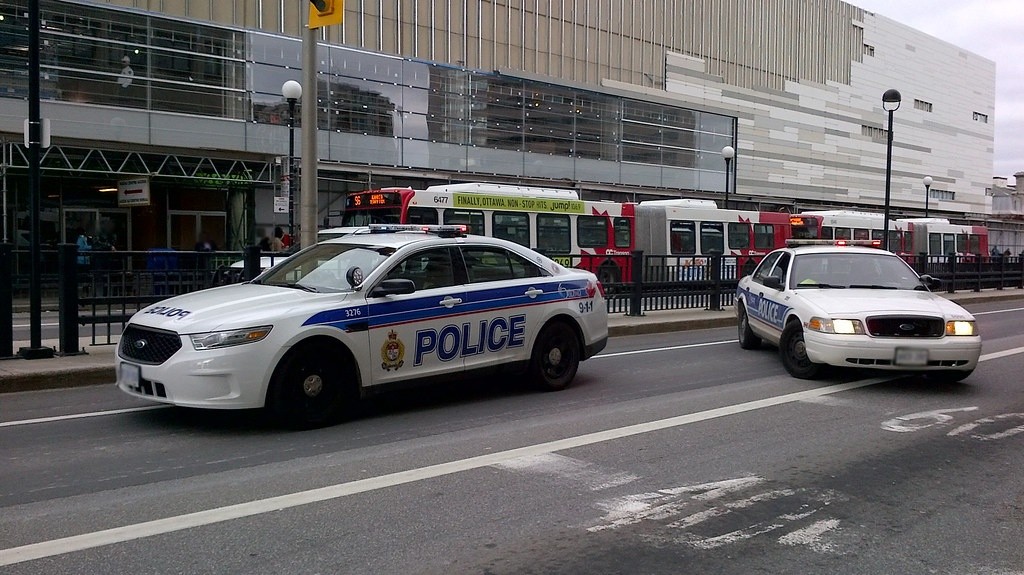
[114,226,610,429]
[733,238,983,381]
[225,227,372,282]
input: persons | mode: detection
[257,225,292,251]
[196,231,225,268]
[242,236,262,281]
[991,245,1024,264]
[114,55,133,109]
[74,224,116,298]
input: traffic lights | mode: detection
[306,1,344,29]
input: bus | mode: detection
[790,207,989,276]
[323,182,793,297]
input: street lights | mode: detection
[283,80,303,247]
[722,145,735,209]
[882,90,903,250]
[922,176,933,217]
